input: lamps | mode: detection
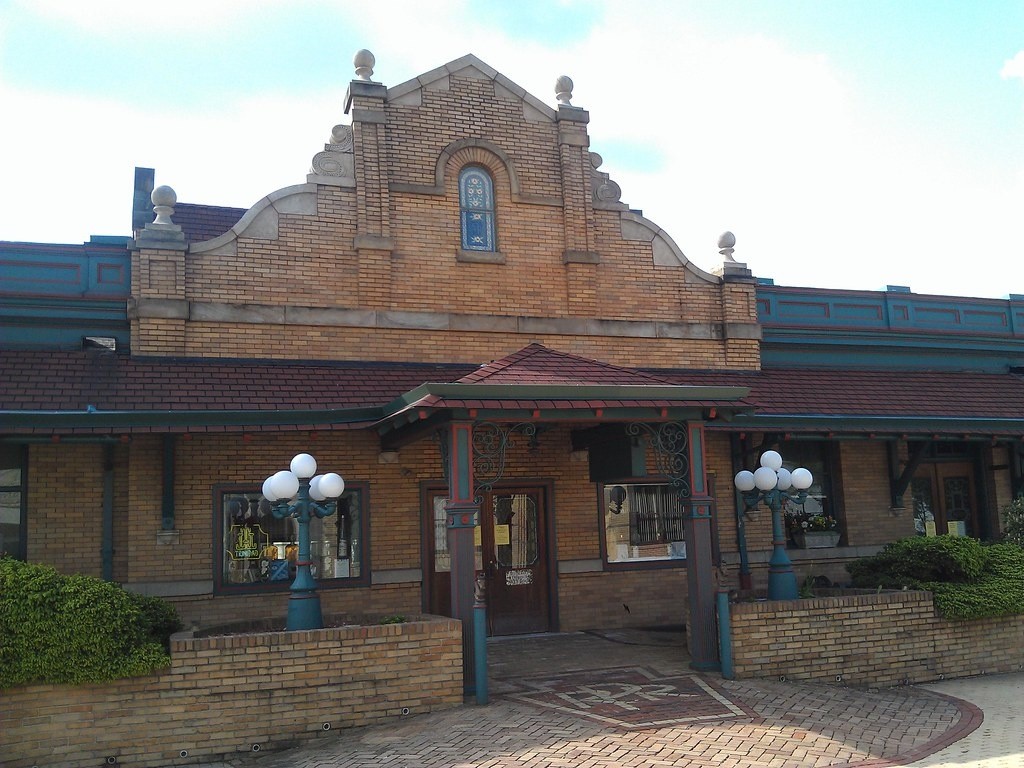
[257,452,346,519]
[734,451,814,509]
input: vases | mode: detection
[792,531,842,549]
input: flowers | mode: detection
[790,515,836,532]
